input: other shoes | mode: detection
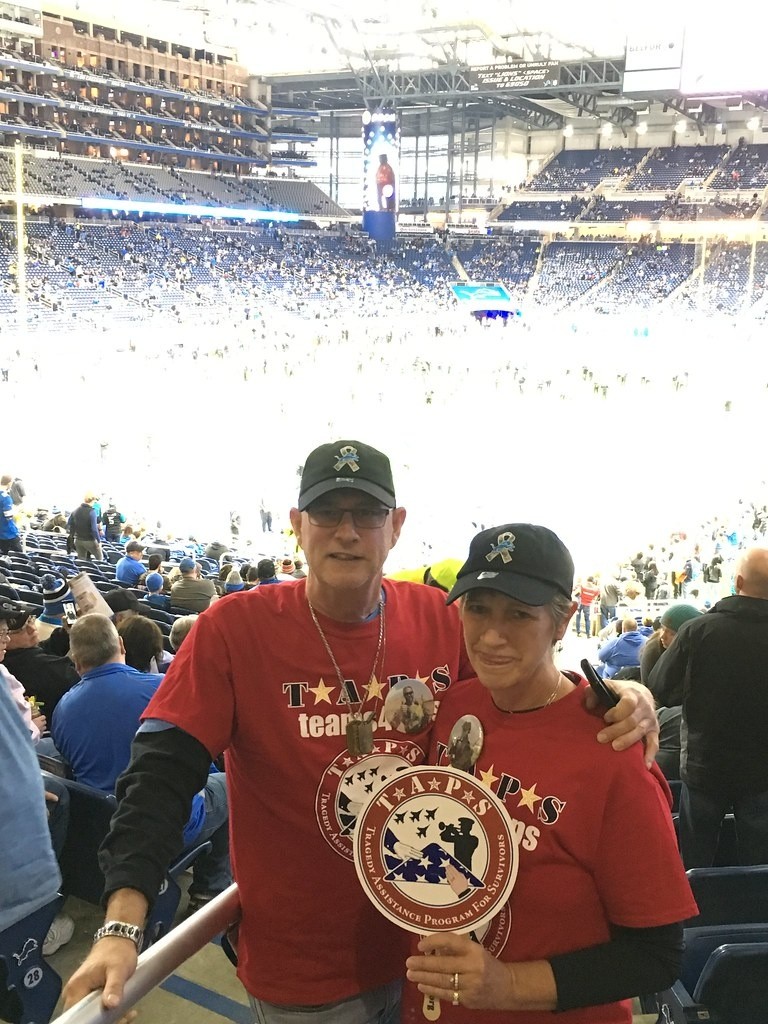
[41,914,75,956]
[186,892,218,915]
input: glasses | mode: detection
[308,505,393,529]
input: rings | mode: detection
[453,992,459,1005]
[450,973,458,990]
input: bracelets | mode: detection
[93,922,144,952]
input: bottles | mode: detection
[31,705,41,719]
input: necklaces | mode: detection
[308,602,386,755]
[339,612,386,753]
[545,674,562,708]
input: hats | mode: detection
[298,440,396,512]
[125,541,145,553]
[179,558,196,572]
[445,524,574,607]
[0,596,45,629]
[661,605,703,632]
[283,560,296,574]
[146,573,164,592]
[224,571,244,592]
[104,589,151,612]
[41,574,74,615]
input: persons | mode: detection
[0,477,767,1023]
[448,722,471,767]
[60,440,660,1024]
[2,8,768,417]
[392,687,432,733]
[406,521,698,1024]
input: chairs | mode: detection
[0,532,240,958]
[635,780,768,1024]
[1,143,768,326]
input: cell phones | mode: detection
[62,600,77,625]
[581,659,619,710]
